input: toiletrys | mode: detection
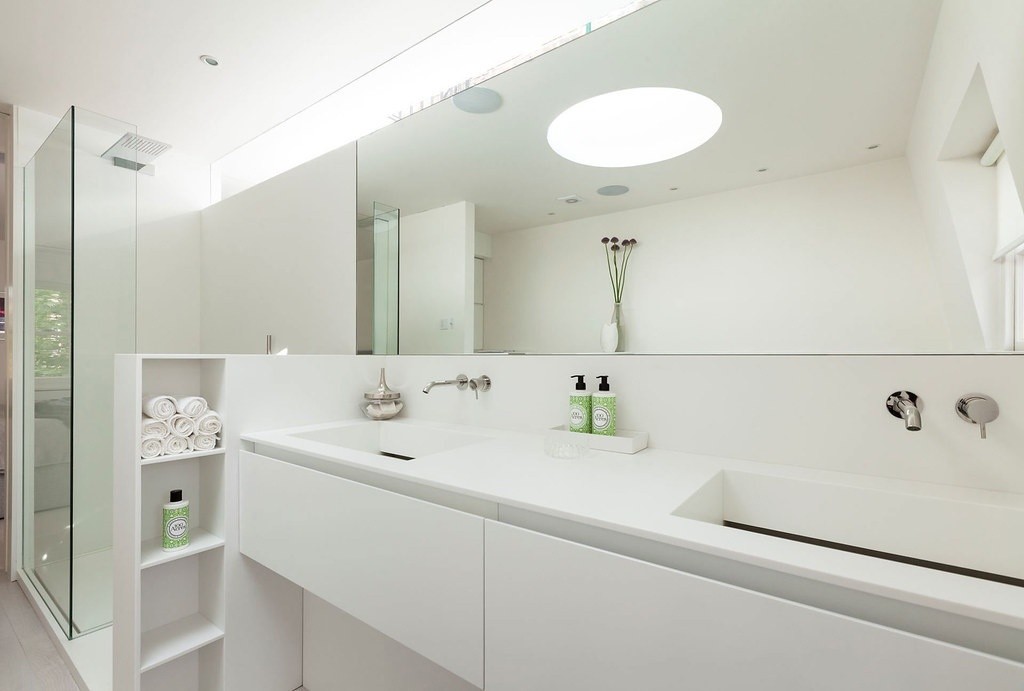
[592,376,616,437]
[568,375,592,435]
[161,489,191,553]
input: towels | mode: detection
[141,394,222,459]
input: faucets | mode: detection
[886,390,922,432]
[422,374,468,395]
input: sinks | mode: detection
[671,468,1023,590]
[287,422,488,462]
